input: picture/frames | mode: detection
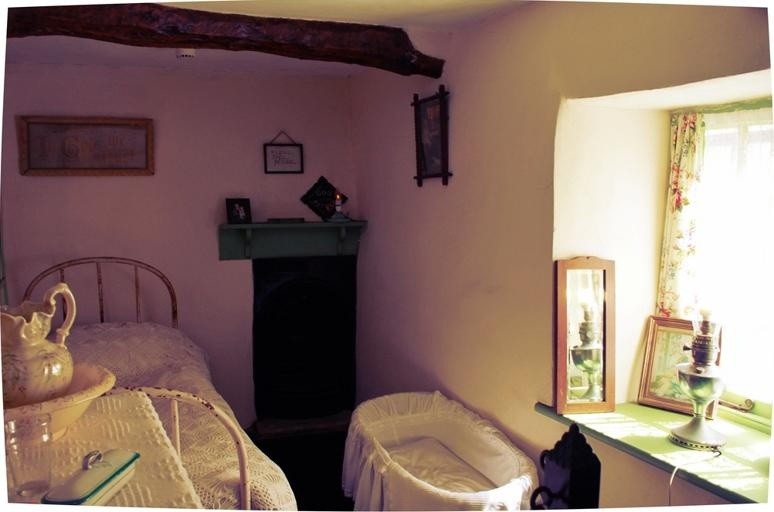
[262,143,303,174]
[13,114,154,178]
[637,314,722,421]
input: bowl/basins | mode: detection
[4,360,117,446]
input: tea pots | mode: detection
[1,281,78,408]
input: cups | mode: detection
[4,412,53,498]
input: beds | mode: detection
[17,255,279,509]
[350,390,538,510]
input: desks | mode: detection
[6,390,203,508]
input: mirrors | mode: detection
[554,256,616,416]
